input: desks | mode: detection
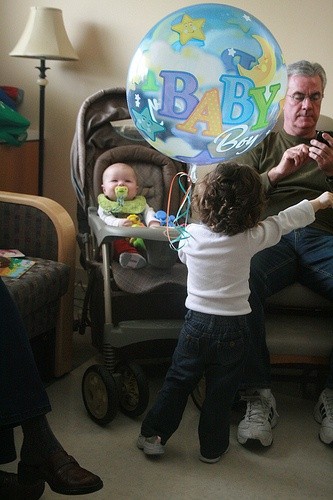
[0,131,46,198]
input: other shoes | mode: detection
[199,444,230,464]
[0,468,45,500]
[19,436,103,495]
[136,427,165,455]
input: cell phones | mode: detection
[313,131,333,149]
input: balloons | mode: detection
[126,4,287,167]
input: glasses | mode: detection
[287,92,325,103]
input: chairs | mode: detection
[261,113,333,366]
[0,192,76,381]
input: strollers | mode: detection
[69,85,208,425]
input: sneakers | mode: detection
[236,389,279,449]
[312,388,333,445]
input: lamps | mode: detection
[6,6,80,196]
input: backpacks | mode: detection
[0,99,32,147]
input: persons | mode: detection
[227,61,333,450]
[137,161,333,463]
[98,163,160,270]
[0,255,103,500]
